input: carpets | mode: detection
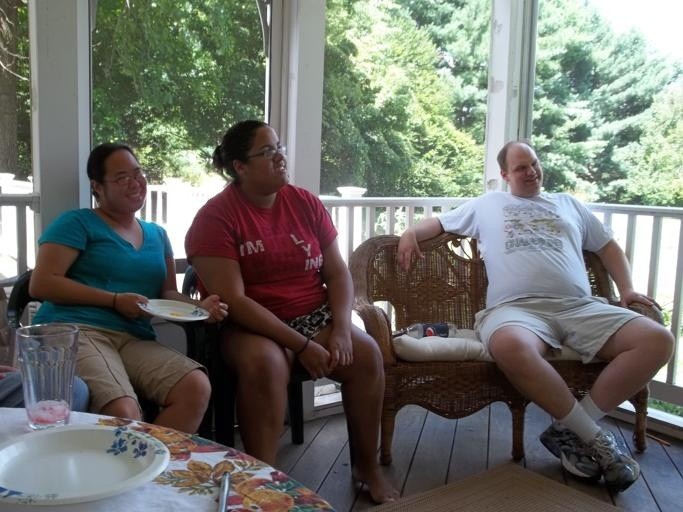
[361,461,622,512]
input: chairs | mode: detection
[2,262,214,437]
[180,256,346,452]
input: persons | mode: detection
[397,141,675,491]
[184,119,401,505]
[29,142,229,435]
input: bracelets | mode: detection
[296,336,310,355]
[112,292,117,310]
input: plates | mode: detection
[137,298,211,323]
[1,422,171,507]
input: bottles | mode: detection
[400,323,458,339]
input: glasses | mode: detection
[234,145,289,162]
[97,169,149,189]
[538,421,604,483]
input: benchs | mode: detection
[346,222,665,466]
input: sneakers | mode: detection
[586,425,641,494]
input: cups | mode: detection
[14,321,80,432]
